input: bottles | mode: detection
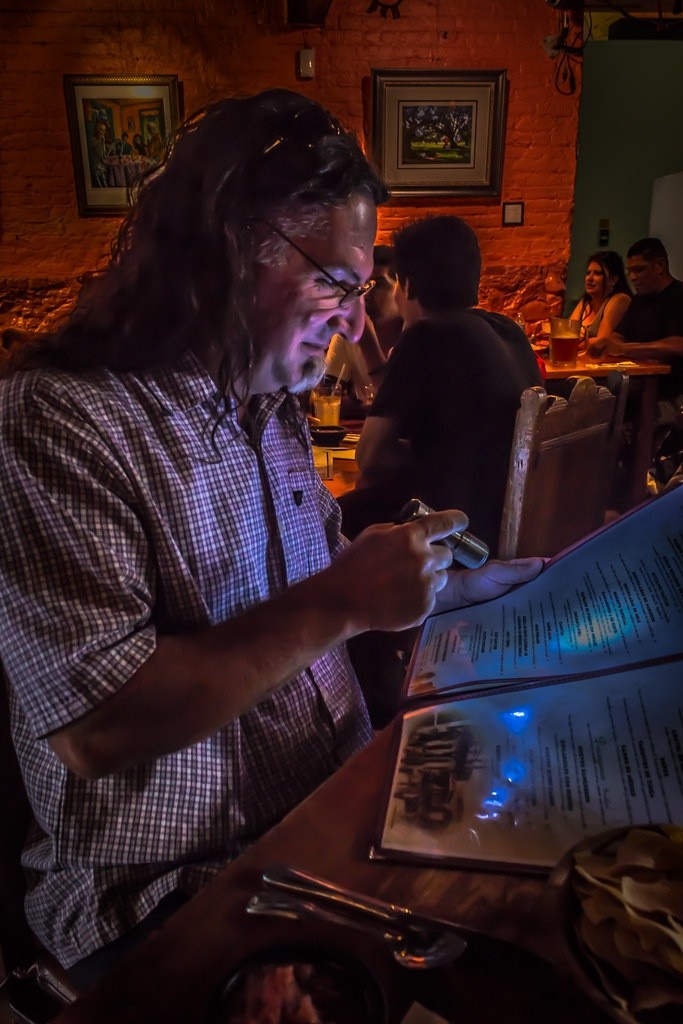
[515,311,527,332]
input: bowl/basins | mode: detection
[310,425,349,446]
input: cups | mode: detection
[311,384,341,426]
[551,319,589,369]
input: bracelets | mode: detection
[368,366,387,376]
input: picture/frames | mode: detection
[63,72,182,217]
[370,67,508,197]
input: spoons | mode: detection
[243,888,468,971]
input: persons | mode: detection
[309,247,404,419]
[0,90,551,1024]
[589,237,683,521]
[336,214,542,723]
[545,253,633,402]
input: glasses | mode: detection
[245,107,343,177]
[242,217,376,310]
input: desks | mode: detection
[307,416,367,504]
[538,351,671,510]
[42,703,683,1024]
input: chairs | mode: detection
[497,370,630,560]
